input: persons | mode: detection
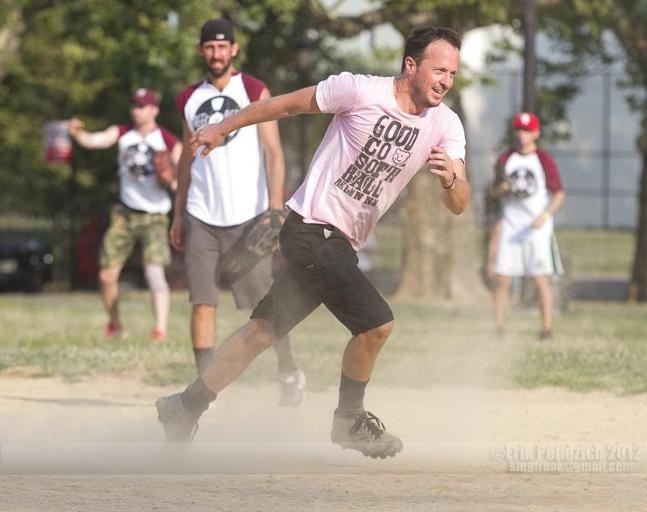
[67,87,184,344]
[353,229,379,294]
[166,18,309,413]
[489,109,568,342]
[153,23,471,461]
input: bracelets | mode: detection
[441,171,458,190]
[542,210,551,222]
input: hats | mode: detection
[128,88,159,107]
[514,112,541,132]
[200,19,234,44]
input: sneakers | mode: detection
[105,323,166,342]
[492,324,555,346]
[279,365,306,407]
[156,391,198,442]
[331,408,403,459]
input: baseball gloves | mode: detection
[152,150,183,190]
[246,208,287,261]
